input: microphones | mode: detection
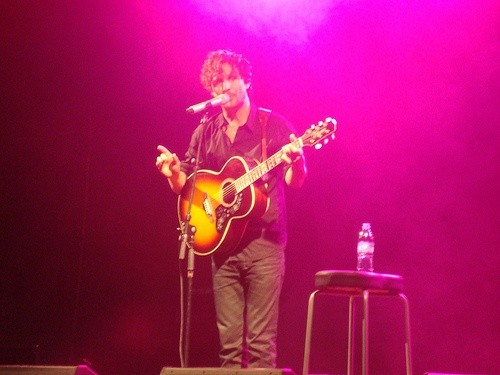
[186,93,231,113]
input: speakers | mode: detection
[0,364,98,375]
[160,367,295,375]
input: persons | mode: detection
[155,50,307,369]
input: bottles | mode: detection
[357,222,375,272]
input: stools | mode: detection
[303,270,411,375]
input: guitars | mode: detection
[177,116,338,257]
